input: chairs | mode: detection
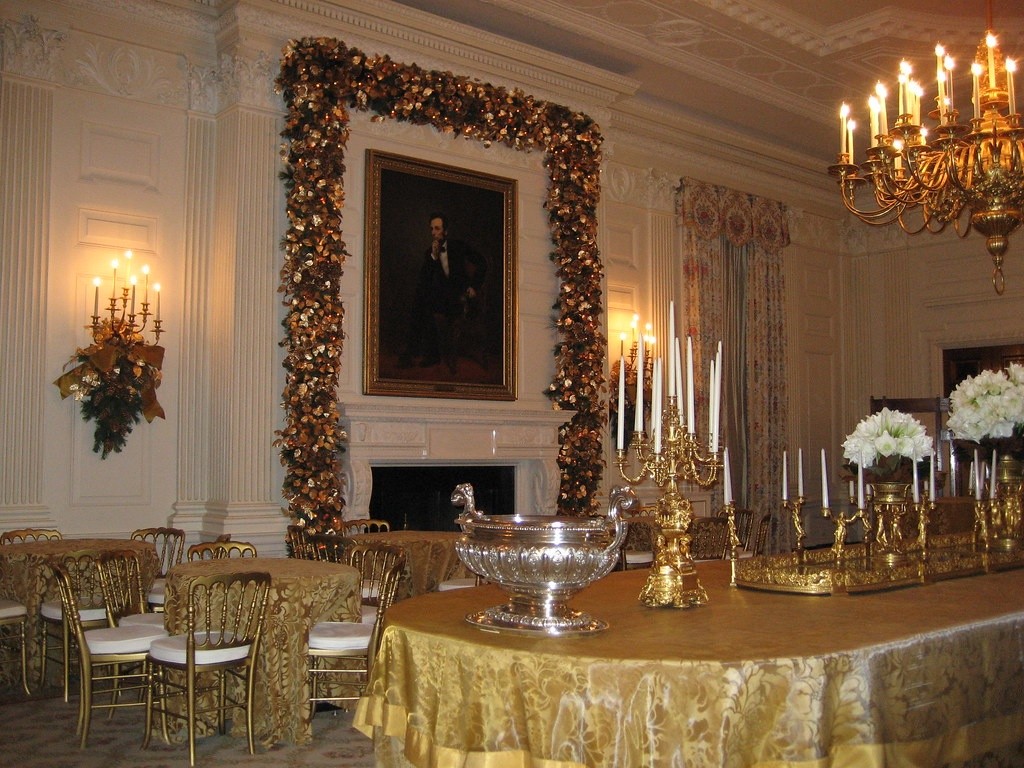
[0,516,412,766]
[613,508,774,570]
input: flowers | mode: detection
[945,362,1024,449]
[834,408,928,481]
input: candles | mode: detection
[614,304,739,503]
[927,450,940,500]
[821,449,829,511]
[795,448,803,496]
[912,451,919,502]
[847,448,875,508]
[961,447,999,498]
[779,449,788,501]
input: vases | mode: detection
[872,481,909,505]
[997,454,1024,497]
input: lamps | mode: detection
[828,0,1024,293]
[601,301,668,417]
[49,248,172,463]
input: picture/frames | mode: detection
[362,149,518,398]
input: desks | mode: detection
[151,563,360,749]
[363,548,1022,767]
[348,528,468,597]
[3,537,157,695]
[3,536,158,689]
